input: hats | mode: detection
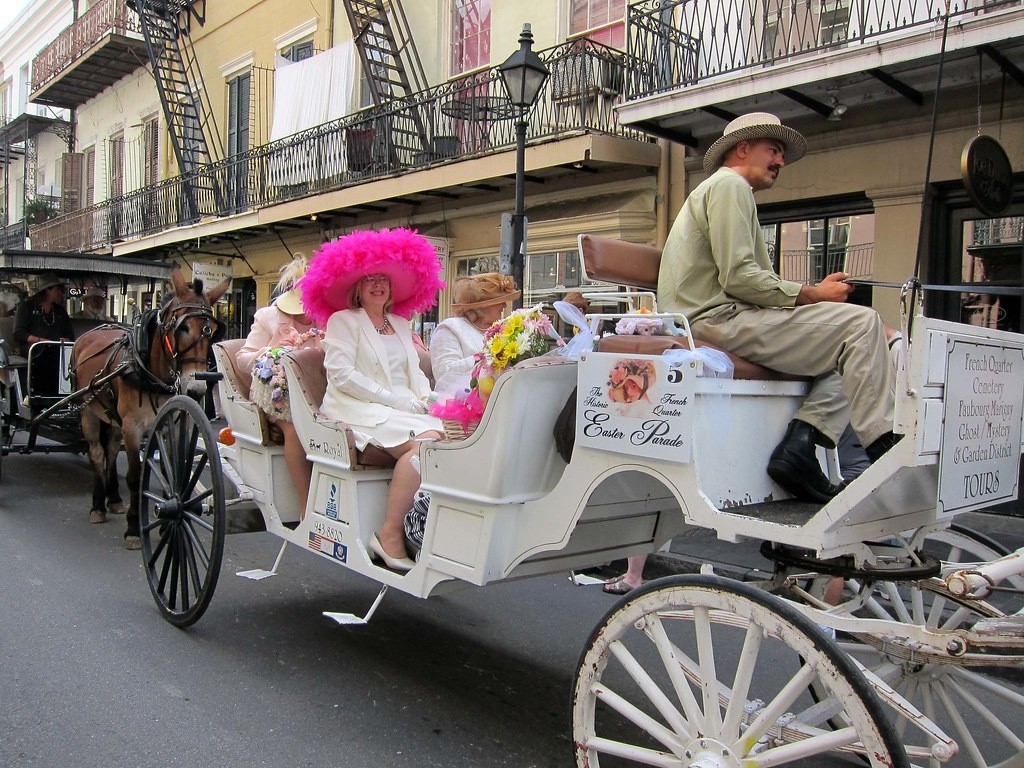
[37,272,69,292]
[703,112,807,175]
[275,253,308,315]
[450,273,522,309]
[301,227,447,329]
[80,287,109,302]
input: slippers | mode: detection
[603,580,634,594]
[605,574,625,584]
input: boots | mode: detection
[767,419,841,499]
[866,432,899,462]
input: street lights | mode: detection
[495,22,551,314]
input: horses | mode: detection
[70,258,235,547]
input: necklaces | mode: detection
[42,304,54,326]
[374,315,390,335]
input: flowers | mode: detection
[251,349,294,409]
[478,300,567,370]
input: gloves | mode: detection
[376,391,430,414]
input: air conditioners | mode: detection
[550,45,619,101]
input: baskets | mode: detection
[252,330,324,418]
[444,309,548,440]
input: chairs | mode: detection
[348,125,382,176]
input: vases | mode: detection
[247,368,291,422]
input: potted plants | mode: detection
[23,200,55,224]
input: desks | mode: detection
[439,93,528,151]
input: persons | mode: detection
[12,273,75,397]
[603,553,648,595]
[319,272,445,569]
[235,275,319,523]
[562,292,592,335]
[429,273,521,383]
[69,287,114,321]
[658,113,903,504]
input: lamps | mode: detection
[310,214,318,221]
[574,163,584,169]
[209,237,218,241]
[822,91,850,123]
[234,234,241,239]
[265,226,276,235]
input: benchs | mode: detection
[418,347,583,508]
[282,343,436,486]
[211,338,285,448]
[576,230,822,401]
[0,315,129,372]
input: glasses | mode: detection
[362,276,390,287]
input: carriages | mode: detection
[0,248,233,553]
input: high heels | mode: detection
[369,532,415,570]
